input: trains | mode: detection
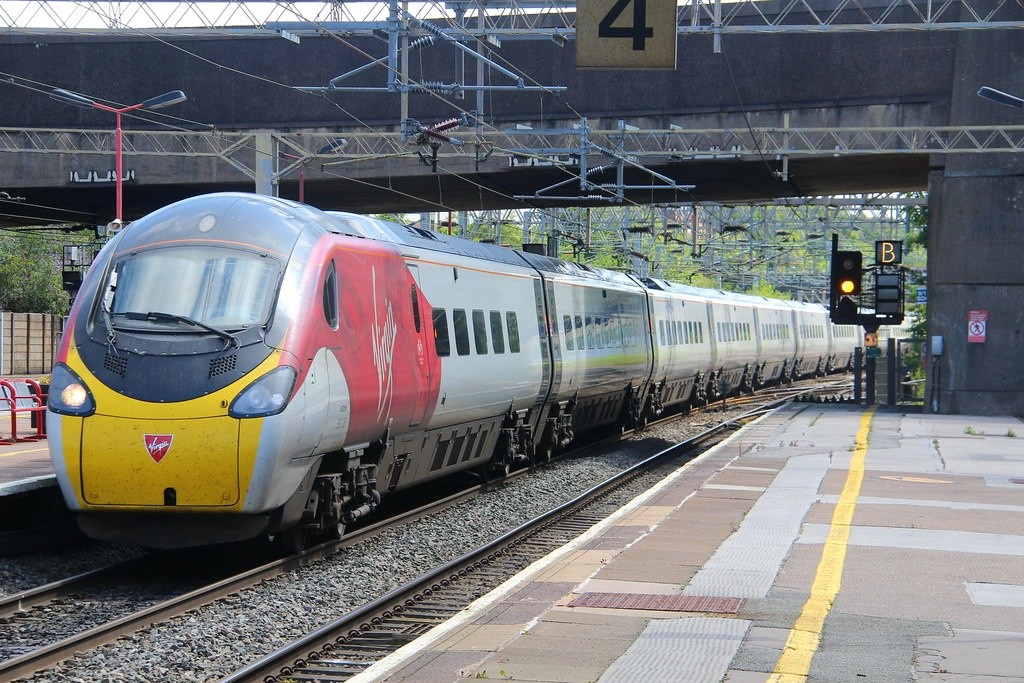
[43,190,927,560]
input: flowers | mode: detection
[38,375,51,385]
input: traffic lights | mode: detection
[835,252,864,328]
[874,272,905,320]
[874,240,906,270]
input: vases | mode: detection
[26,384,49,434]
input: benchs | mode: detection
[0,378,49,445]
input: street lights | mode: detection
[50,86,188,219]
